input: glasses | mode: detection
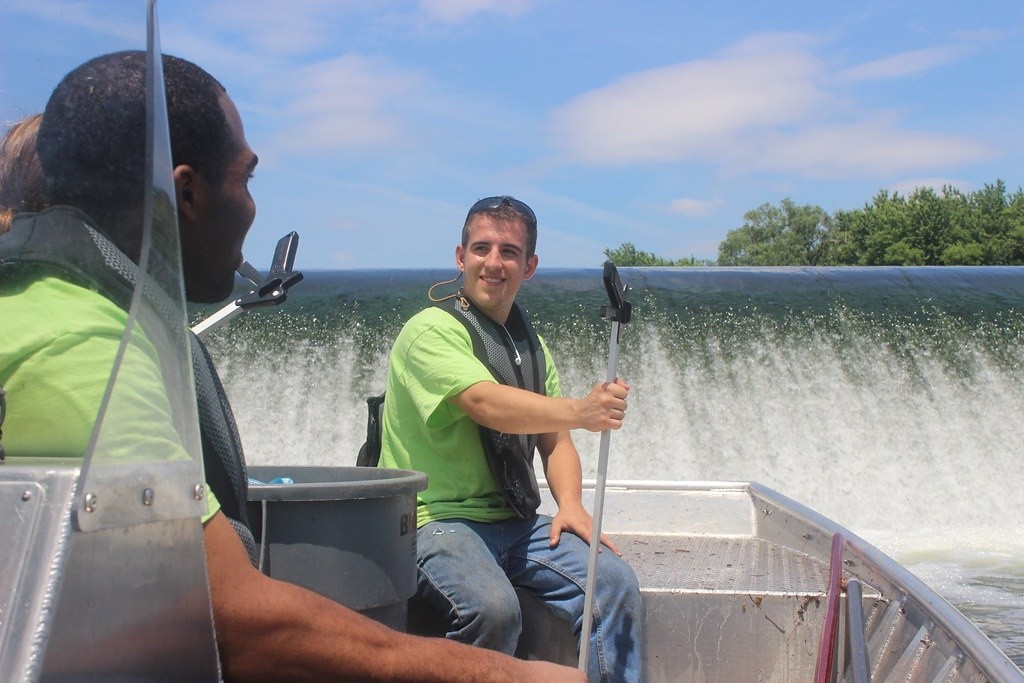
[464,197,537,247]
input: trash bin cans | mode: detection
[233,466,427,634]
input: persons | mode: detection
[0,54,591,683]
[376,196,644,683]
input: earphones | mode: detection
[515,350,522,366]
